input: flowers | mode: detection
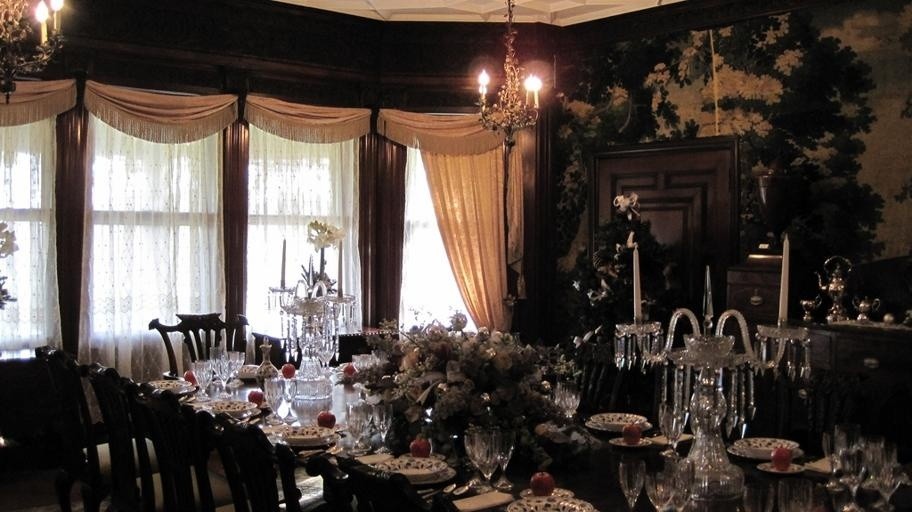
[352,315,592,459]
[309,220,342,248]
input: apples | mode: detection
[183,370,196,386]
[344,362,355,377]
[248,390,263,407]
[410,435,431,458]
[530,471,554,496]
[771,444,791,471]
[282,363,295,378]
[622,421,641,445]
[317,407,336,429]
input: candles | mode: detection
[282,241,286,289]
[779,234,790,320]
[40,19,49,47]
[338,240,343,298]
[632,241,642,323]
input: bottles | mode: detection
[256,336,278,393]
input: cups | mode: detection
[742,477,813,511]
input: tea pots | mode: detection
[799,256,880,324]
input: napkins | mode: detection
[190,403,202,409]
[354,451,394,466]
[452,489,517,512]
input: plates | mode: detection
[204,400,257,418]
[148,379,192,394]
[521,487,575,502]
[377,456,457,485]
[284,426,333,444]
[586,412,653,448]
[191,347,245,401]
[727,437,805,474]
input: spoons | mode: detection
[422,483,469,507]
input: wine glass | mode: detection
[319,349,335,374]
[464,427,514,494]
[822,423,905,511]
[344,403,396,456]
[264,378,298,423]
[618,406,695,512]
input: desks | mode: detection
[150,359,911,511]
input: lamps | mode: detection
[0,1,68,107]
[474,0,542,154]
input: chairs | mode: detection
[148,312,250,368]
[37,347,160,510]
[113,376,235,511]
[305,450,433,509]
[191,409,325,511]
[567,312,911,466]
